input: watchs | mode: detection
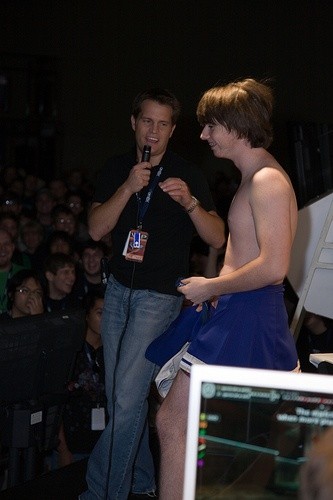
[184,196,200,214]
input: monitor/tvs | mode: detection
[182,365,333,500]
[0,306,86,412]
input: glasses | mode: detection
[15,288,41,297]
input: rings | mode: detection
[179,184,184,191]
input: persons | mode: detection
[0,160,333,500]
[145,78,301,500]
[78,86,225,500]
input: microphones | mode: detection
[136,145,152,199]
